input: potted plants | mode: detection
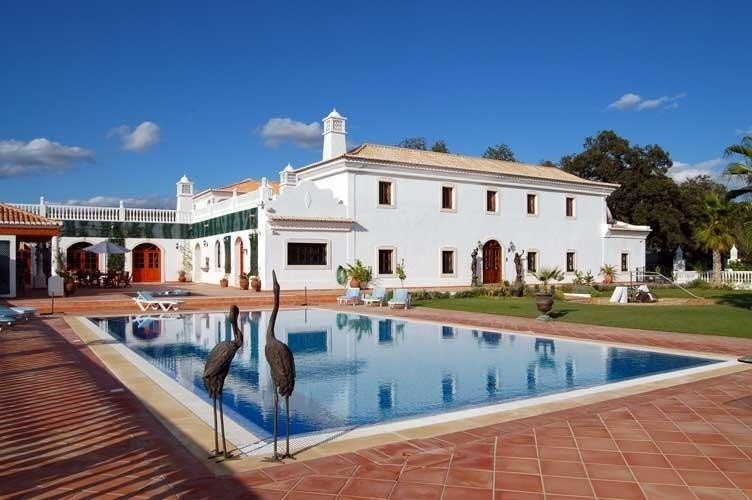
[343,259,372,290]
[240,312,261,323]
[348,314,372,342]
[178,270,186,282]
[220,274,228,287]
[597,264,621,281]
[238,272,260,291]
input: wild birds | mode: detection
[201,304,246,464]
[262,268,298,463]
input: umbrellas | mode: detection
[83,238,132,278]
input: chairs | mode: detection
[0,306,36,332]
[132,291,186,311]
[125,314,176,329]
[73,268,136,289]
[336,287,411,312]
